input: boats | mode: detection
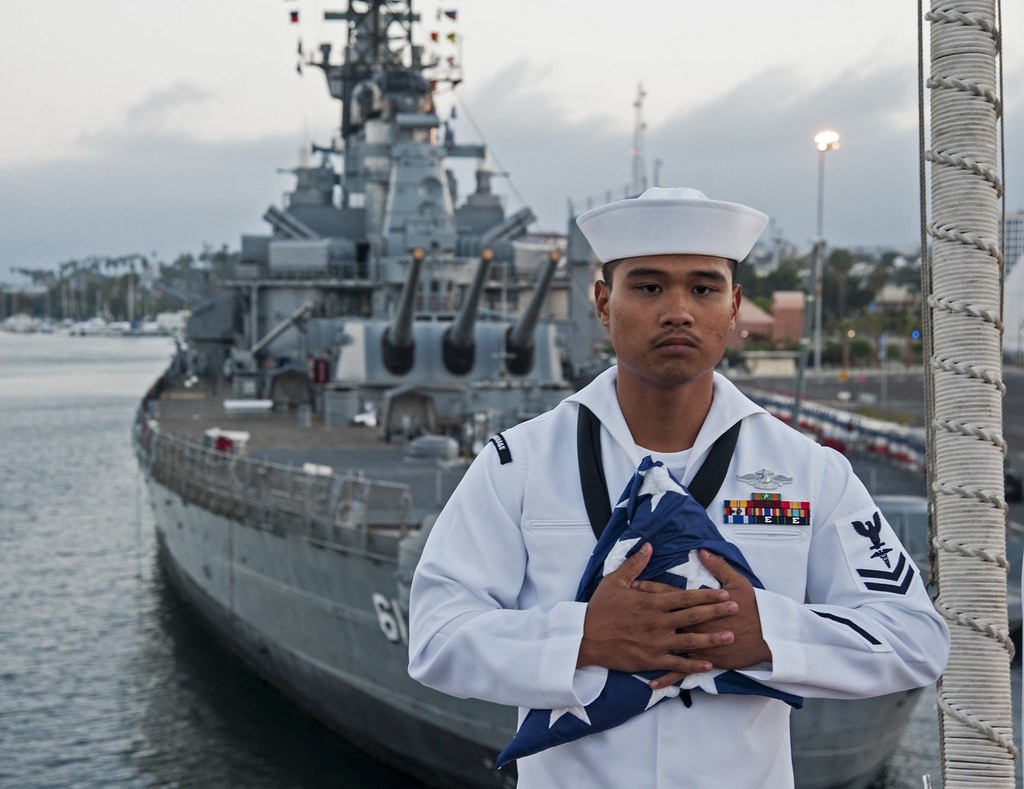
[7,310,188,342]
[131,0,941,789]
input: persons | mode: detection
[408,187,950,789]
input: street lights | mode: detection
[809,127,840,370]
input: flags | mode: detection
[496,455,804,770]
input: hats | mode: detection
[577,189,771,265]
